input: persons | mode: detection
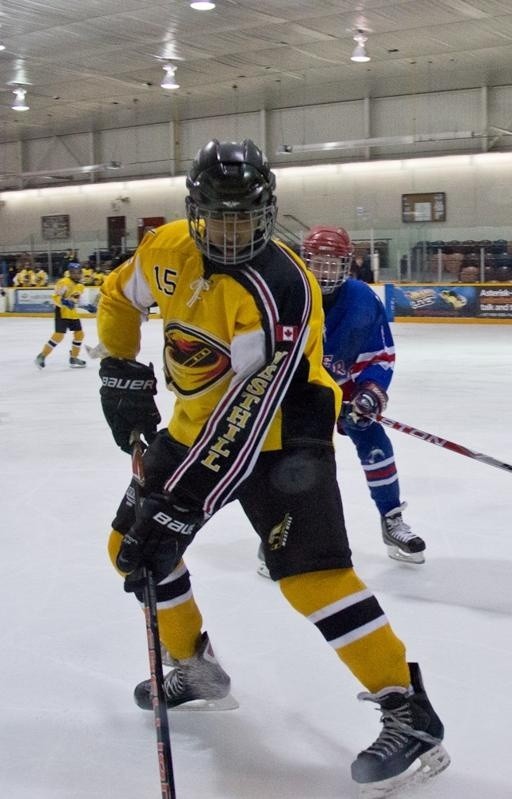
[35,261,87,369]
[257,224,426,556]
[1,247,108,288]
[353,255,373,282]
[94,138,442,788]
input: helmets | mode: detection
[184,138,276,265]
[69,263,82,271]
[297,225,353,297]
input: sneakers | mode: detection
[69,356,86,366]
[381,506,426,557]
[36,353,45,368]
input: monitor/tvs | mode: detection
[42,215,70,240]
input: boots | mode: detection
[350,659,446,784]
[133,631,233,710]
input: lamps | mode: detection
[191,0,216,10]
[161,62,180,89]
[11,86,29,112]
[351,32,371,63]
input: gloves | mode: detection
[63,299,74,310]
[96,355,161,457]
[336,380,389,437]
[116,485,205,595]
[85,304,96,314]
